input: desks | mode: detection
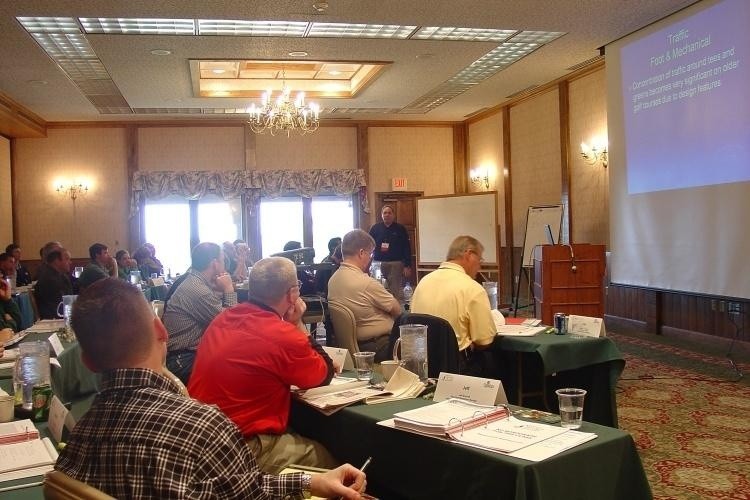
[337,366,632,500]
[496,318,611,409]
[15,280,41,327]
[0,315,71,500]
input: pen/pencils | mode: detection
[340,457,371,500]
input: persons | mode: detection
[284,241,314,296]
[163,242,237,387]
[53,277,367,500]
[368,205,412,302]
[408,236,498,376]
[5,244,32,288]
[0,253,16,280]
[40,241,63,261]
[185,256,337,476]
[327,229,402,365]
[219,241,247,282]
[131,245,152,281]
[0,269,23,333]
[233,239,244,251]
[315,244,342,293]
[108,250,141,283]
[321,237,342,263]
[35,248,73,320]
[77,243,118,290]
[144,243,164,278]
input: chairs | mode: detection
[322,301,363,368]
[387,311,463,376]
[42,471,116,500]
[302,292,328,343]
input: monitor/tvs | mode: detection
[545,224,556,246]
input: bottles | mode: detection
[554,313,565,335]
[404,283,412,310]
[32,383,53,422]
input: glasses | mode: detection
[290,279,303,291]
[465,248,485,264]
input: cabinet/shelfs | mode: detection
[376,187,425,285]
[529,242,609,327]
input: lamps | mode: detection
[578,130,607,168]
[470,165,495,189]
[249,67,321,138]
[52,174,90,201]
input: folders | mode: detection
[376,397,598,462]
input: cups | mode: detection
[6,275,17,296]
[554,388,587,430]
[151,273,158,281]
[56,295,80,342]
[74,267,83,279]
[482,282,498,311]
[14,341,52,412]
[153,303,165,318]
[126,270,141,286]
[393,324,428,385]
[353,351,376,380]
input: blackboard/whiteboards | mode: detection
[522,203,564,267]
[415,191,499,266]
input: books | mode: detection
[393,397,570,454]
[0,419,59,473]
[490,318,547,337]
[291,375,393,417]
